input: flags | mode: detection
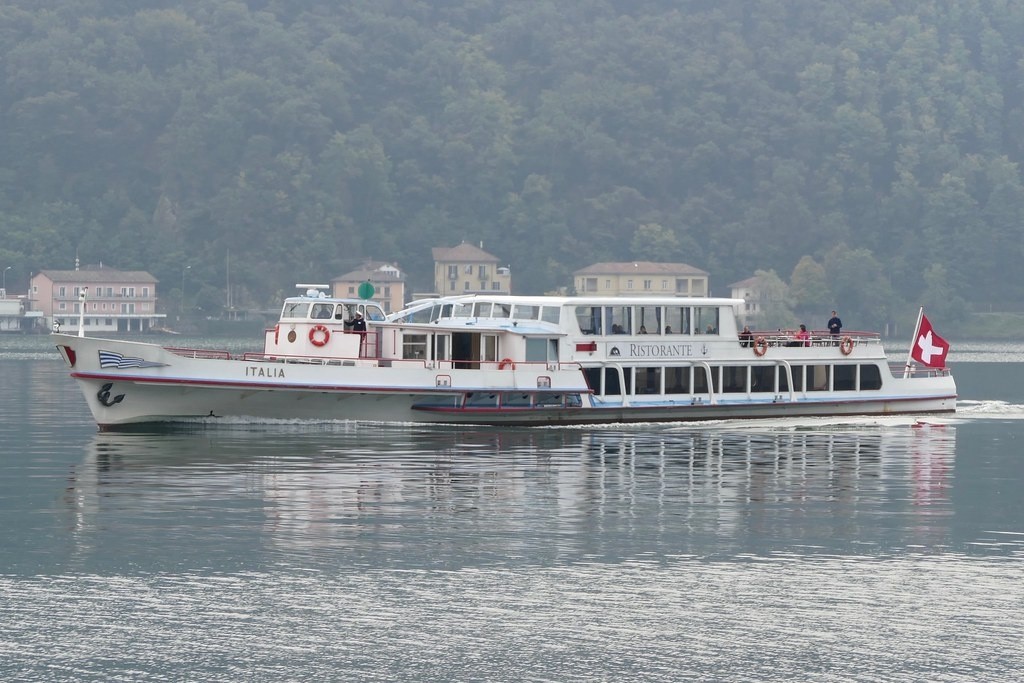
[911,314,949,371]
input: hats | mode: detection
[356,311,362,316]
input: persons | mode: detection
[828,311,842,347]
[790,324,810,347]
[639,326,648,334]
[344,311,366,358]
[666,325,673,334]
[706,325,714,334]
[611,324,626,334]
[739,326,754,347]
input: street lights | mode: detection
[181,266,191,314]
[3,266,12,287]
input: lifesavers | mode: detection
[753,336,768,357]
[309,325,329,346]
[274,323,279,345]
[498,358,516,370]
[840,336,854,355]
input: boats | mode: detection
[47,283,958,433]
